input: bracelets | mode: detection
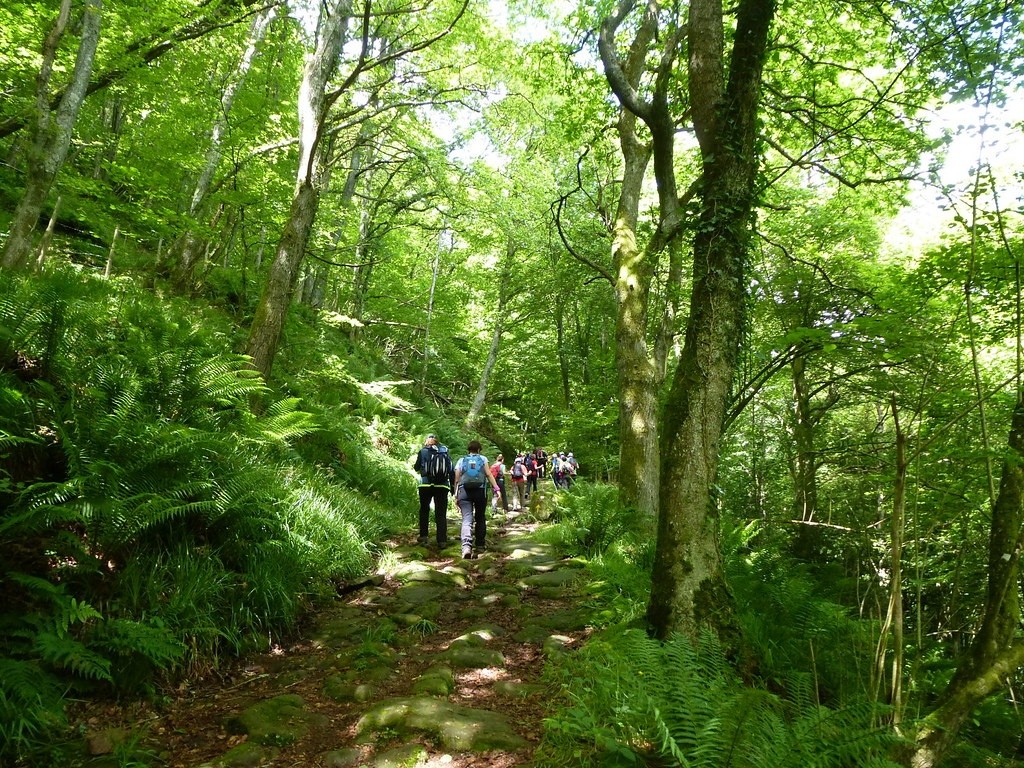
[494,486,500,492]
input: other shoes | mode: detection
[492,508,497,515]
[462,548,471,559]
[512,506,517,511]
[438,542,446,549]
[477,546,484,552]
[525,493,528,499]
[417,535,427,542]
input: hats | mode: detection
[568,453,574,457]
[515,458,522,462]
[552,454,557,458]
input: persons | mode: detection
[454,440,502,559]
[412,435,455,549]
[510,447,580,513]
[490,454,510,516]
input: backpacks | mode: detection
[537,450,543,459]
[427,445,452,484]
[513,463,522,478]
[525,461,533,472]
[490,464,502,479]
[462,455,484,478]
